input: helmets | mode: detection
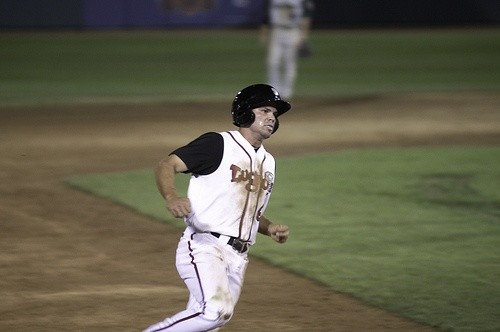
[233,83,290,132]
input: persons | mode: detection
[260,0,314,101]
[143,84,294,332]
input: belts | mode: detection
[210,232,247,254]
